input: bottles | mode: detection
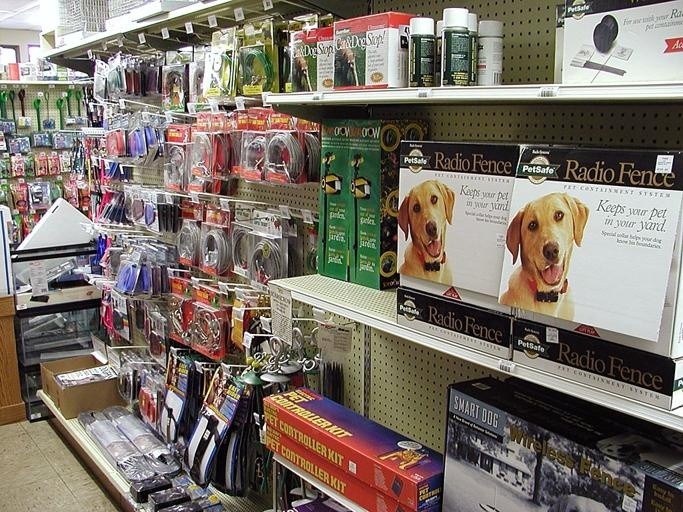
[409,7,504,86]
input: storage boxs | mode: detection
[286,25,336,93]
[315,115,432,293]
[503,142,683,416]
[262,385,443,511]
[398,136,527,362]
[334,10,419,89]
[550,0,683,87]
[442,370,683,512]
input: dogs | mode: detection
[397,179,456,287]
[499,192,590,322]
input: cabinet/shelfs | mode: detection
[262,0,683,512]
